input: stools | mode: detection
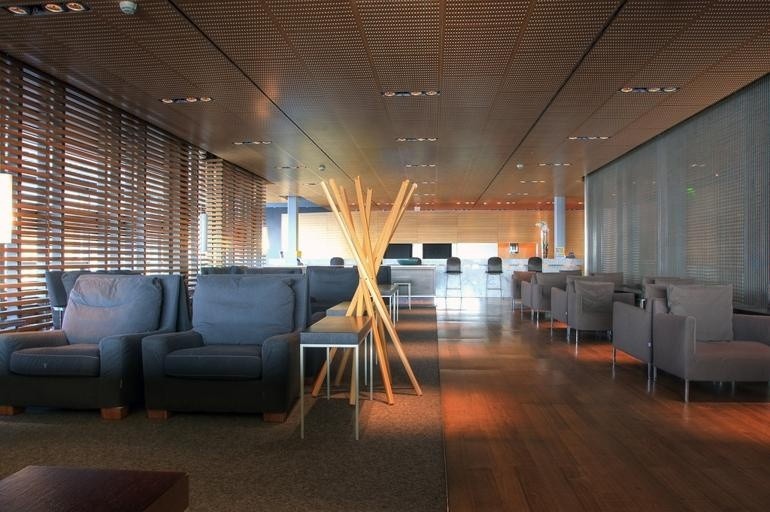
[394,279,413,312]
[299,315,373,441]
[326,302,379,386]
[369,284,398,327]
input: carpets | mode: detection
[2,295,449,512]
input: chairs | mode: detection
[142,273,308,423]
[529,257,542,272]
[612,279,671,392]
[0,273,181,421]
[486,257,503,301]
[170,255,392,382]
[512,269,657,375]
[651,282,770,402]
[444,257,462,300]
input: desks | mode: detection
[1,464,191,510]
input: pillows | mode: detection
[665,282,736,342]
